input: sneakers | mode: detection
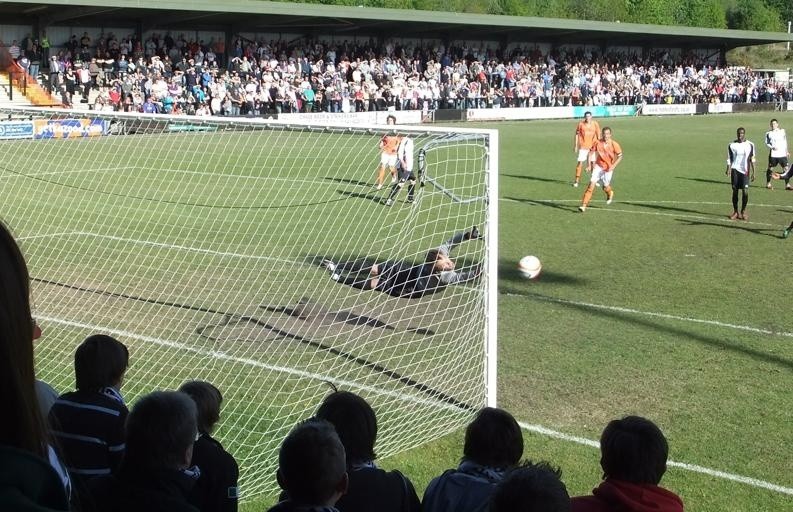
[317,257,341,282]
[783,225,790,238]
[375,176,398,190]
[578,206,584,212]
[606,190,614,204]
[573,182,601,187]
[730,212,749,220]
[785,184,793,190]
[385,198,415,206]
[766,182,774,189]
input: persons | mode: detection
[375,116,400,193]
[768,162,792,240]
[571,109,602,188]
[1,216,78,509]
[43,333,135,494]
[419,404,524,511]
[764,118,792,192]
[577,125,623,213]
[311,380,422,510]
[0,26,793,112]
[725,126,757,221]
[566,412,685,512]
[315,225,482,300]
[31,322,75,421]
[385,134,417,206]
[86,392,206,511]
[263,418,348,511]
[484,457,572,512]
[171,379,239,511]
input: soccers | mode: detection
[517,255,542,280]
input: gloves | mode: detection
[472,225,484,241]
